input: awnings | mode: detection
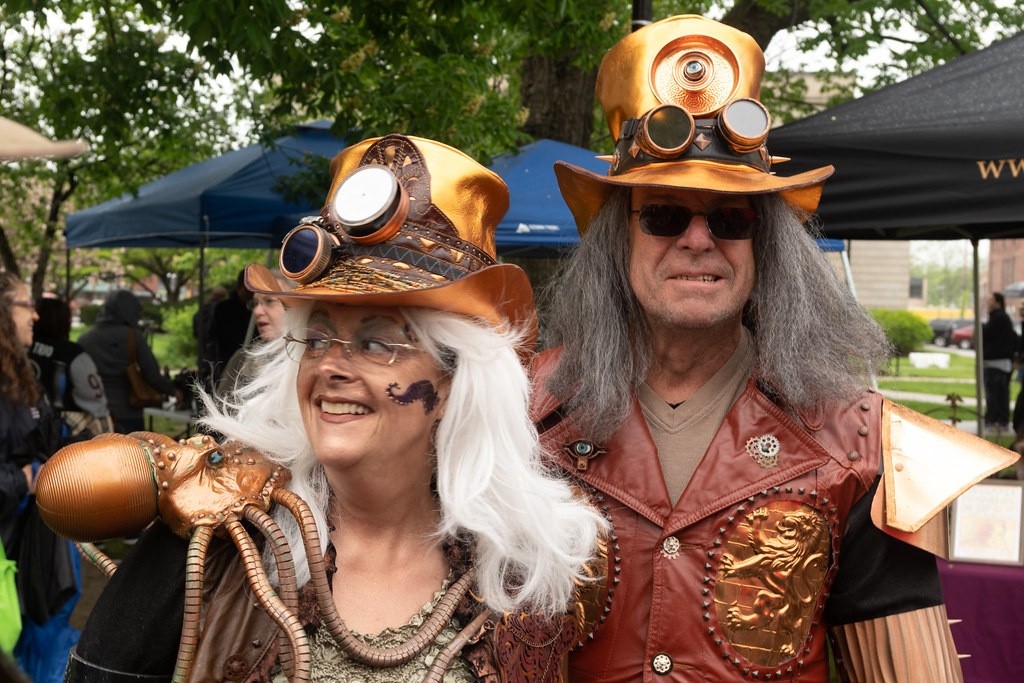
[38,135,566,683]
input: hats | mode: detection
[554,14,835,240]
[245,133,539,365]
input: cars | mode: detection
[931,317,975,348]
[950,324,975,349]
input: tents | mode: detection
[766,28,1024,440]
[476,140,845,260]
[0,114,91,157]
[64,119,392,309]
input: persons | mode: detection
[0,267,78,683]
[24,267,306,472]
[980,293,1024,452]
[519,15,1024,683]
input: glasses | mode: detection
[246,297,281,310]
[283,326,456,369]
[624,205,756,240]
[9,299,36,312]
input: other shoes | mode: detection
[984,423,1010,435]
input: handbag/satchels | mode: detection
[124,330,166,408]
